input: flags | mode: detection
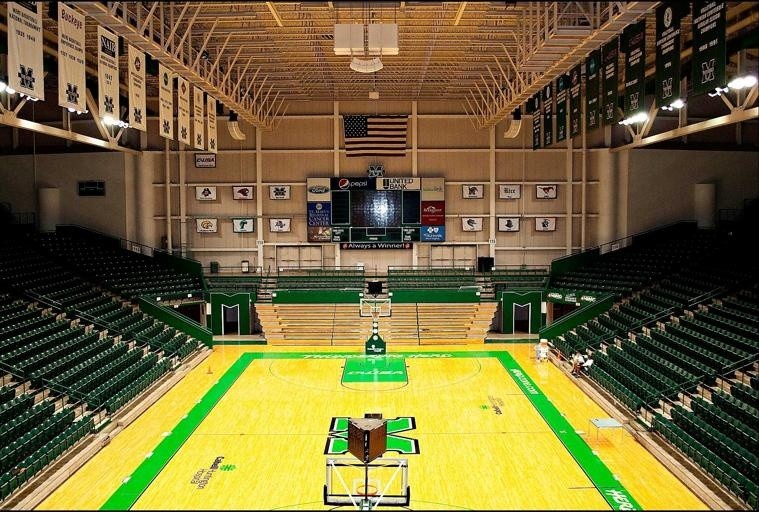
[6,0,220,156]
[530,1,728,151]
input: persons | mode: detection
[575,355,594,378]
[571,350,586,375]
[535,336,549,365]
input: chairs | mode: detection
[214,266,540,304]
[3,203,212,510]
[538,204,757,510]
